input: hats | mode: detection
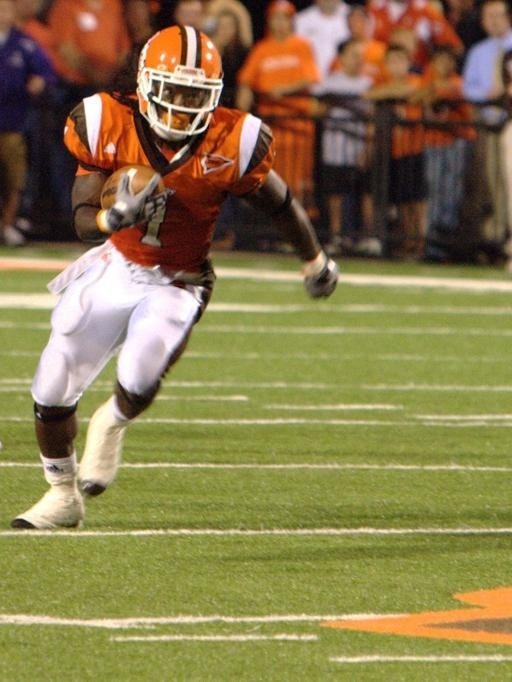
[265,0,298,18]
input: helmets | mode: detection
[136,24,225,142]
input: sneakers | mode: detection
[77,395,133,496]
[322,234,512,270]
[2,214,34,251]
[253,236,297,255]
[9,452,85,529]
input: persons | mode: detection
[10,24,339,531]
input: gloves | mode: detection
[299,249,342,302]
[96,170,176,236]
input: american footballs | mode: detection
[101,165,164,208]
